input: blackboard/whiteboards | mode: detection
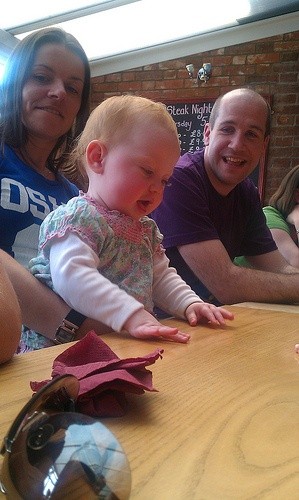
[157,93,272,208]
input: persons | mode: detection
[0,263,21,365]
[16,95,234,354]
[146,88,299,320]
[233,164,299,269]
[0,27,115,353]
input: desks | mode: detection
[0,302,299,500]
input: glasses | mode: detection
[0,373,131,500]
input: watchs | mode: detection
[53,309,86,345]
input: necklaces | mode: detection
[38,168,52,177]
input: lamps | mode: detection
[186,62,212,84]
